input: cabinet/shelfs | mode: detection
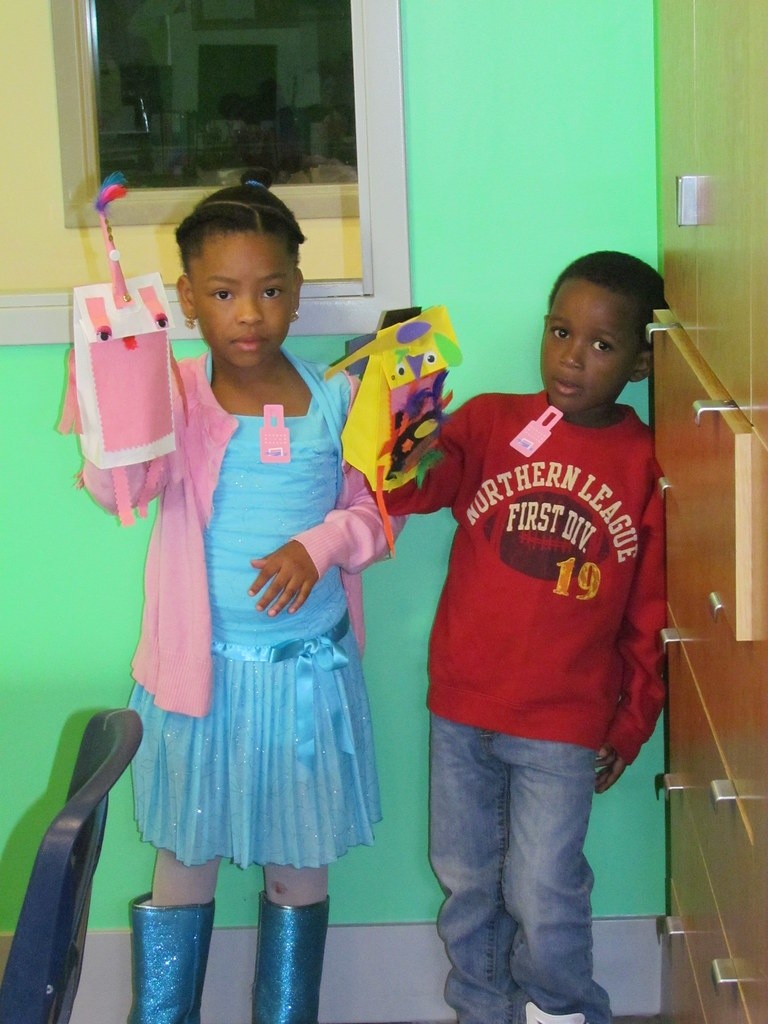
[654,0,767,1022]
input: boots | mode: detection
[251,890,329,1023]
[127,892,217,1024]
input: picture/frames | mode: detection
[0,0,411,346]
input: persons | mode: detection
[360,248,673,1024]
[74,169,408,1023]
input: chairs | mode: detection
[0,708,143,1023]
